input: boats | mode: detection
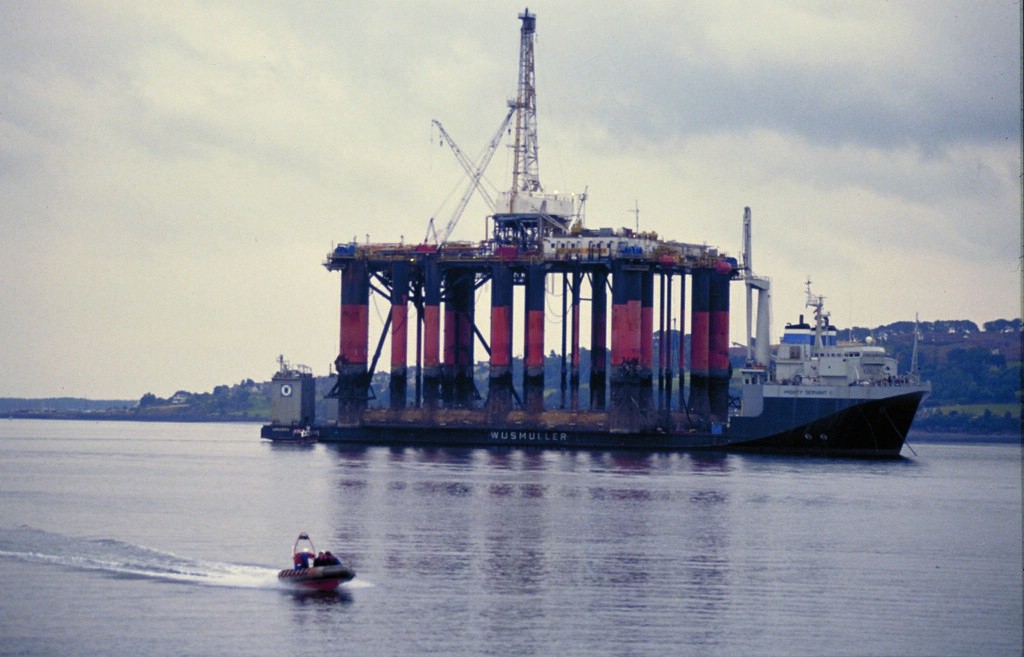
[652,204,933,457]
[292,424,319,443]
[277,533,357,594]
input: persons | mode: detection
[314,551,336,566]
